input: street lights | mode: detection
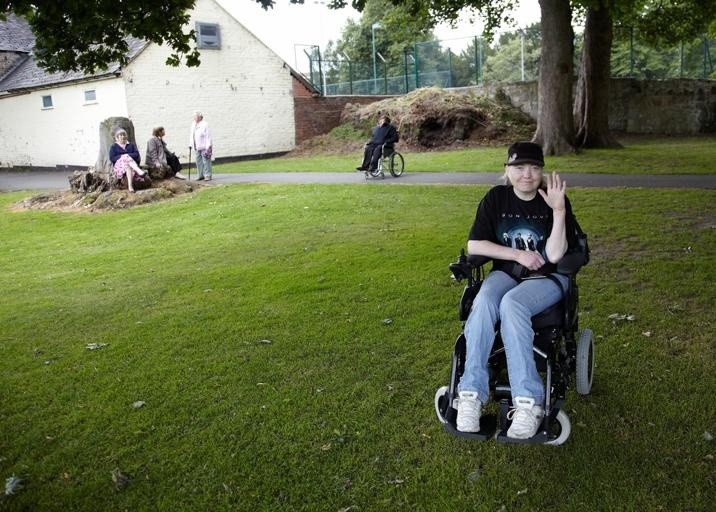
[371,23,383,96]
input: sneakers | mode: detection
[506,396,544,439]
[456,391,481,432]
[174,173,186,179]
[196,176,212,181]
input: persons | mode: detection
[191,111,212,181]
[356,115,399,171]
[457,141,589,440]
[146,126,186,180]
[109,128,147,193]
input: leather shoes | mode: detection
[357,165,374,171]
[127,186,136,193]
[137,169,147,177]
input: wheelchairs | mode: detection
[435,230,601,452]
[360,128,406,181]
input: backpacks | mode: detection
[166,151,182,172]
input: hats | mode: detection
[504,141,544,166]
